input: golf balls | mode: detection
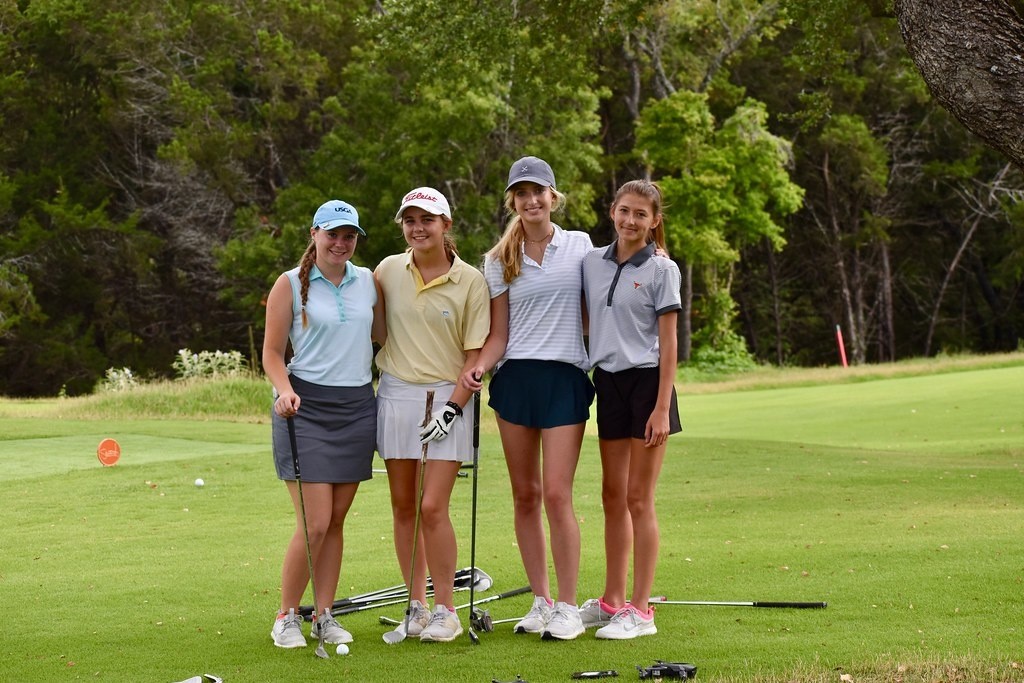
[194,478,205,488]
[336,643,350,655]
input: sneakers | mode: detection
[595,603,657,640]
[420,605,463,642]
[514,595,555,633]
[311,608,353,645]
[271,607,307,649]
[579,597,625,629]
[393,600,431,638]
[541,602,585,641]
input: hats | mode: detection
[504,155,556,194]
[394,185,452,224]
[313,200,366,236]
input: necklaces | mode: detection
[320,269,329,279]
[524,228,554,254]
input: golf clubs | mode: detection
[481,596,669,634]
[380,390,436,646]
[625,599,829,611]
[298,565,494,624]
[285,408,332,662]
[377,585,533,628]
[368,461,480,479]
[467,372,483,645]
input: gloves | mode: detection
[417,401,463,445]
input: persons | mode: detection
[461,155,595,641]
[371,185,491,642]
[262,197,388,650]
[580,180,682,640]
